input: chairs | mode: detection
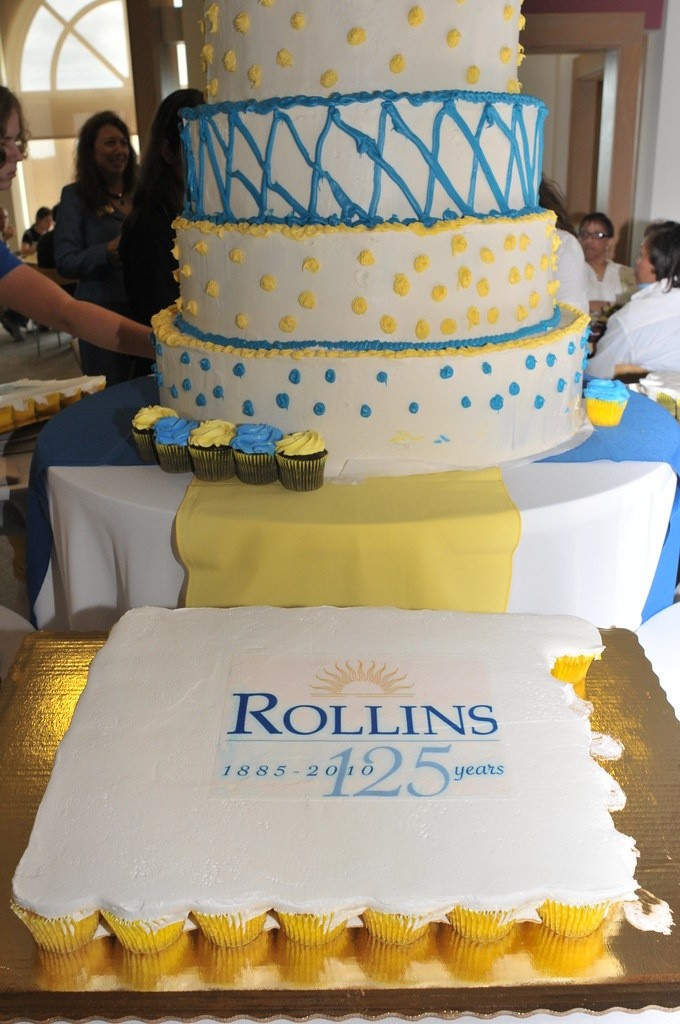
[42,268,78,284]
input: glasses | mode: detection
[579,231,610,240]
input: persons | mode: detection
[0,86,208,385]
[539,171,680,381]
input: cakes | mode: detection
[0,375,106,428]
[150,0,590,480]
[8,601,640,956]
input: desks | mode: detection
[17,251,39,267]
[25,373,680,632]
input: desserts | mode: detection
[130,404,329,491]
[583,378,630,427]
